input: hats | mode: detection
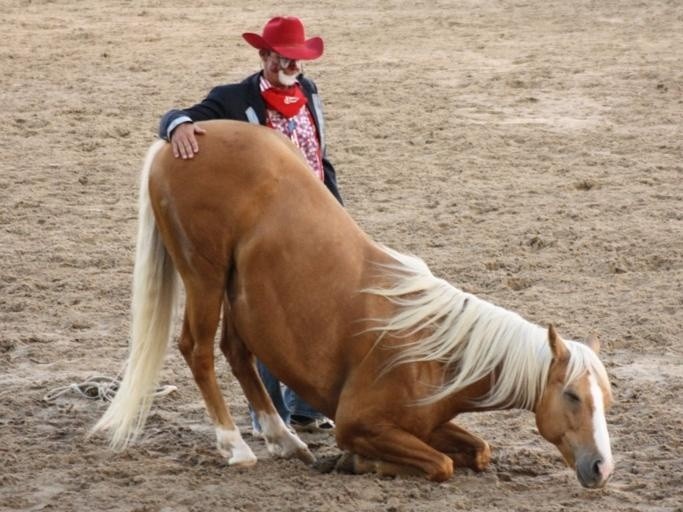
[242,16,323,60]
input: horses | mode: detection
[86,117,616,490]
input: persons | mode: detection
[155,11,343,441]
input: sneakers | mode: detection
[252,426,297,437]
[290,418,334,433]
